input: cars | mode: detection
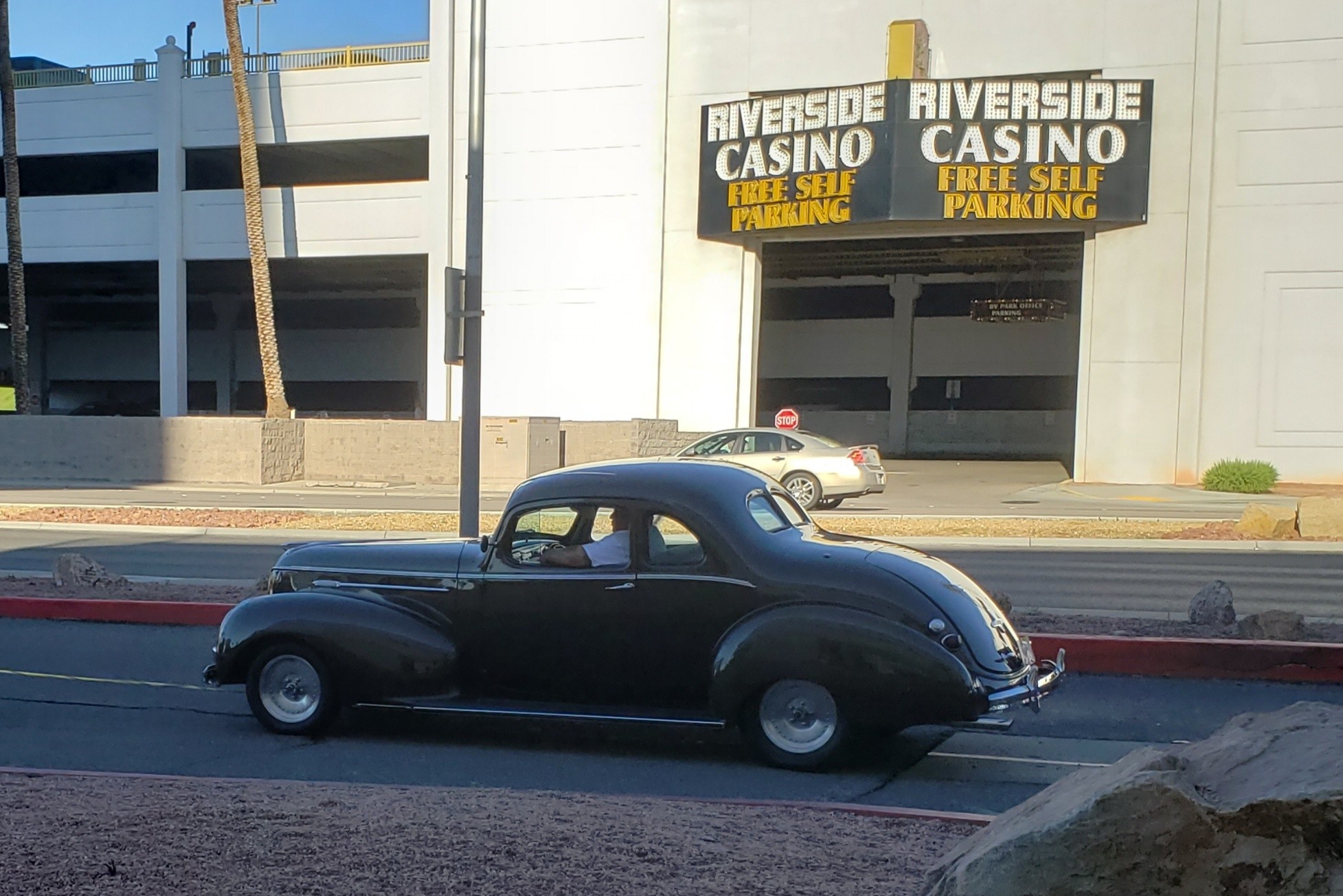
[201,463,1065,774]
[527,427,887,511]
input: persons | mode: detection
[540,505,668,572]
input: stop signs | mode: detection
[774,409,798,429]
